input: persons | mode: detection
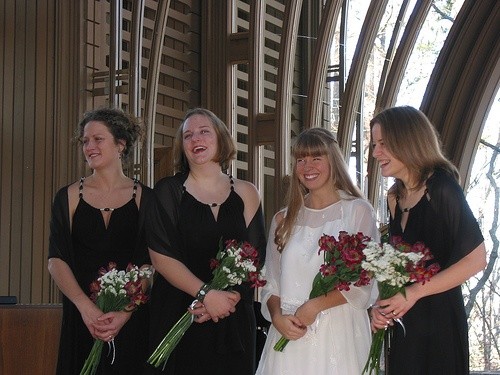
[142,108,266,375]
[369,106,487,375]
[47,109,152,375]
[255,128,381,375]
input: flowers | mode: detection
[274,232,377,353]
[143,238,267,371]
[78,262,159,375]
[359,235,440,374]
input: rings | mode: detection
[392,311,397,316]
[108,335,112,338]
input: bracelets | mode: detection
[196,284,211,303]
